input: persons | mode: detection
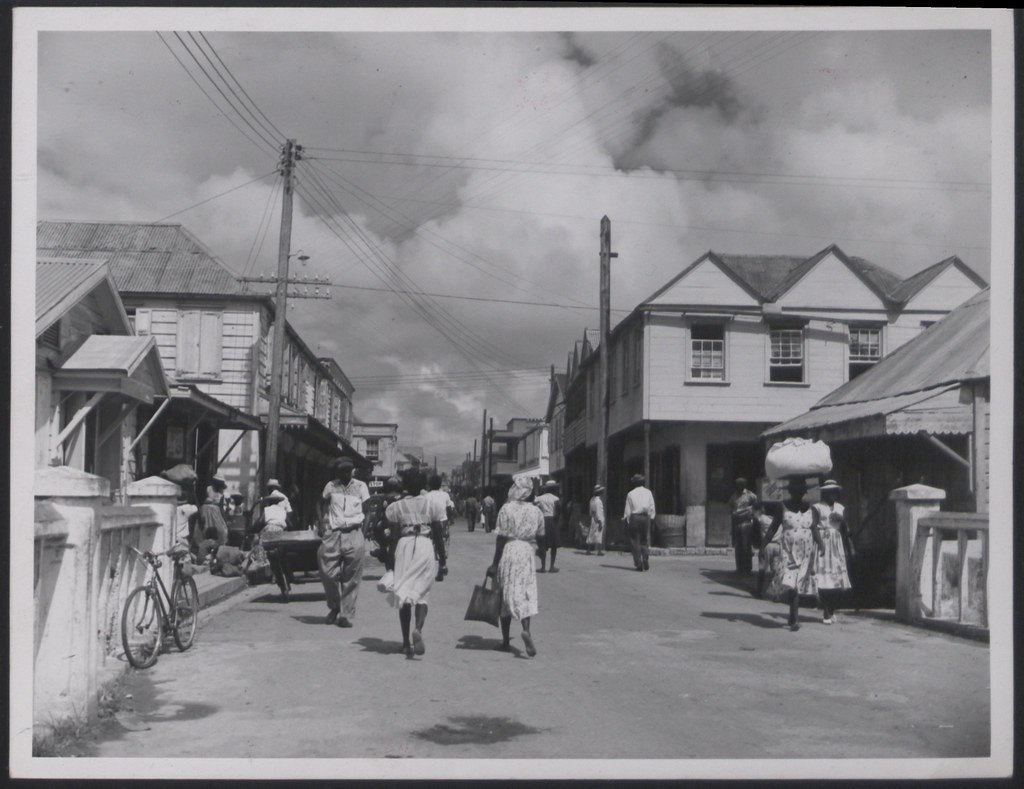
[486,474,546,658]
[621,474,655,572]
[534,480,563,573]
[725,477,855,632]
[175,476,293,584]
[315,457,370,628]
[465,492,500,533]
[374,465,455,658]
[566,484,606,556]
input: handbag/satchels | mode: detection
[376,568,394,593]
[464,574,501,627]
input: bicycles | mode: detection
[121,542,200,669]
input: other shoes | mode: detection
[402,645,410,653]
[536,568,546,573]
[522,631,536,657]
[550,566,559,572]
[443,567,449,576]
[326,608,340,624]
[413,629,425,655]
[790,623,799,632]
[494,644,511,651]
[338,617,353,628]
[637,561,649,570]
[788,616,793,626]
[823,618,832,624]
[831,614,837,623]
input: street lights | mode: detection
[261,248,310,509]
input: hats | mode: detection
[265,478,281,489]
[631,474,645,482]
[541,480,560,488]
[816,479,841,490]
[334,456,354,469]
[213,473,225,481]
[593,484,604,493]
[230,491,246,500]
[263,493,285,501]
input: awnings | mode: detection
[754,381,977,467]
